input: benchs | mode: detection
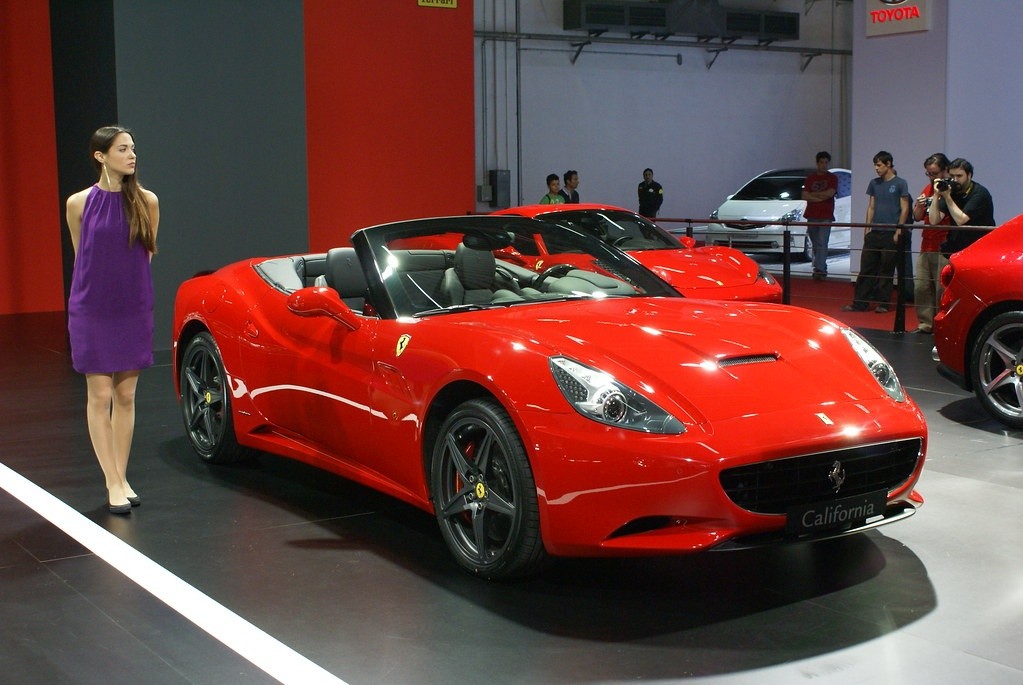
[294,249,456,310]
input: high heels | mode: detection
[106,486,131,513]
[127,495,141,505]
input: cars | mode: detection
[706,167,852,258]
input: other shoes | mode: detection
[813,272,826,280]
[918,323,932,333]
[875,306,887,312]
[840,304,868,312]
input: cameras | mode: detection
[936,177,956,193]
[925,197,933,214]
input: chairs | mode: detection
[325,248,366,313]
[441,242,522,308]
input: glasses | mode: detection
[925,168,946,176]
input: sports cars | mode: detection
[383,202,783,309]
[171,214,931,590]
[938,211,1023,435]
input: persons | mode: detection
[841,150,908,313]
[538,174,565,205]
[909,152,956,335]
[801,151,839,279]
[878,169,916,309]
[557,169,580,205]
[637,168,664,222]
[927,158,997,366]
[66,126,160,515]
